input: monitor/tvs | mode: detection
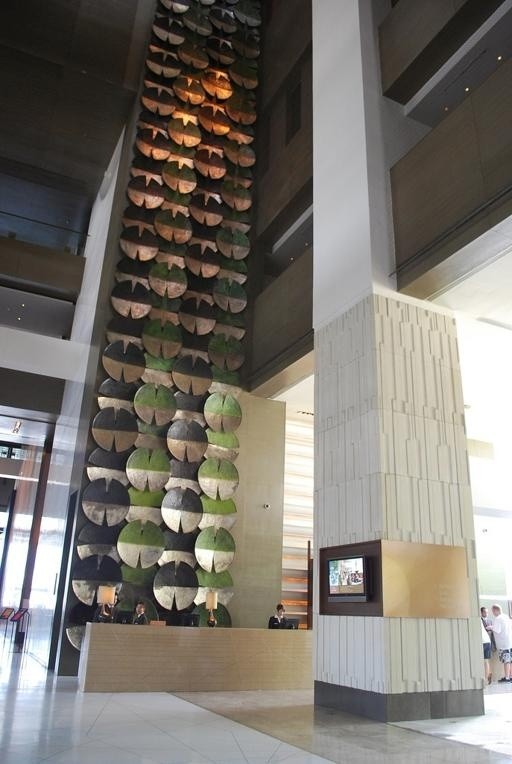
[327,555,370,602]
[174,613,200,627]
[281,617,299,629]
[113,608,135,624]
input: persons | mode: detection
[132,602,147,624]
[486,604,512,683]
[481,620,492,685]
[269,605,288,629]
[94,593,119,623]
[481,607,491,625]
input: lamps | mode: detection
[96,586,116,622]
[206,592,217,628]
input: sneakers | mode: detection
[498,677,512,684]
[488,673,492,685]
